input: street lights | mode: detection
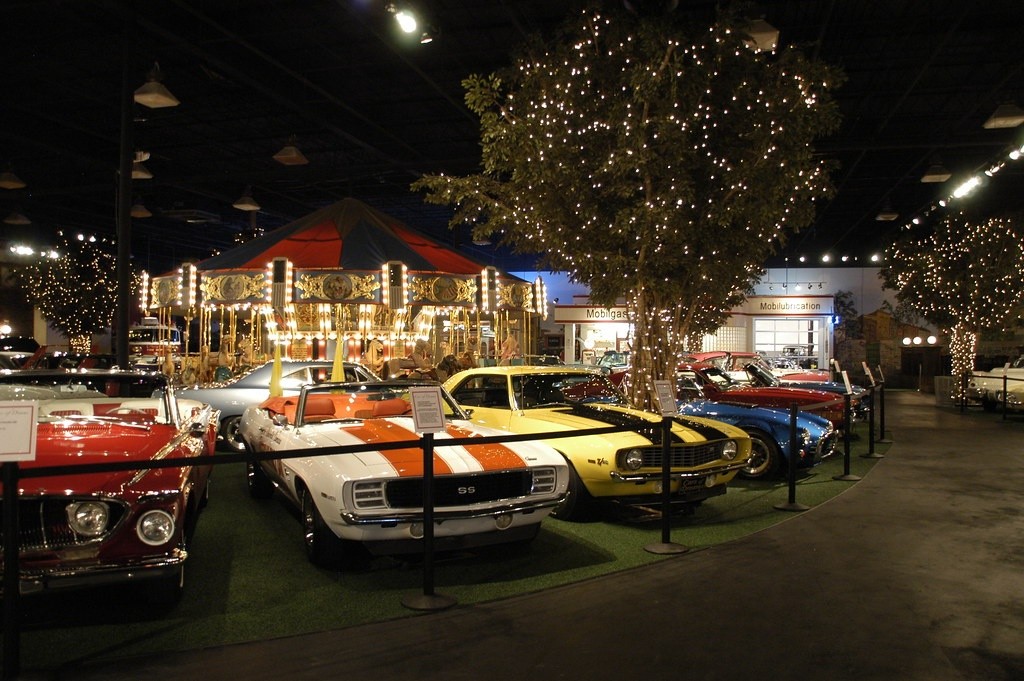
[117,46,182,368]
[238,131,309,240]
[231,185,260,239]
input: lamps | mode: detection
[553,298,559,305]
[769,283,823,291]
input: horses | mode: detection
[162,334,256,388]
[355,336,526,380]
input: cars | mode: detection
[0,331,170,421]
[175,359,401,452]
[968,354,1024,412]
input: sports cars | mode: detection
[554,349,879,479]
[0,400,221,600]
[237,380,572,570]
[442,364,755,522]
